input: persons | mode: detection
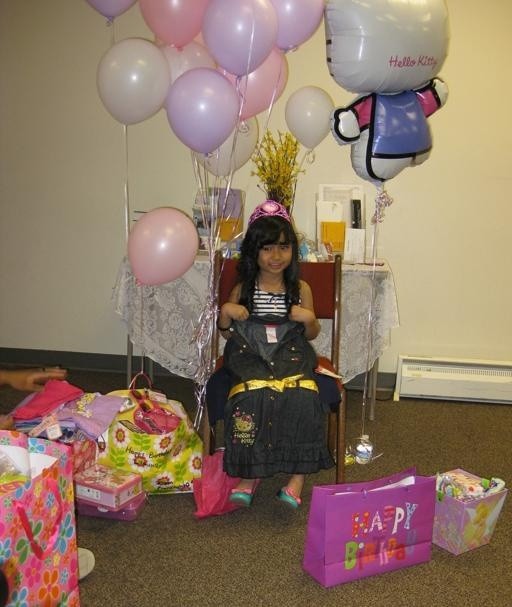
[215,201,334,511]
[0,365,67,430]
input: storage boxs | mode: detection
[432,467,509,557]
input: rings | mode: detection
[43,368,45,372]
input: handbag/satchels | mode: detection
[303,466,437,589]
[95,372,205,496]
[1,430,81,606]
[192,449,242,518]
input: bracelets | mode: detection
[216,322,232,332]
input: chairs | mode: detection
[204,249,346,484]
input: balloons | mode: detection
[88,1,325,177]
[131,207,199,288]
[284,85,334,151]
[324,0,451,190]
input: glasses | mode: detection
[132,381,151,414]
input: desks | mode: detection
[111,257,400,421]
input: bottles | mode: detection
[356,435,373,465]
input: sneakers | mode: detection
[75,546,95,580]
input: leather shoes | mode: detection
[276,484,302,511]
[229,478,260,509]
